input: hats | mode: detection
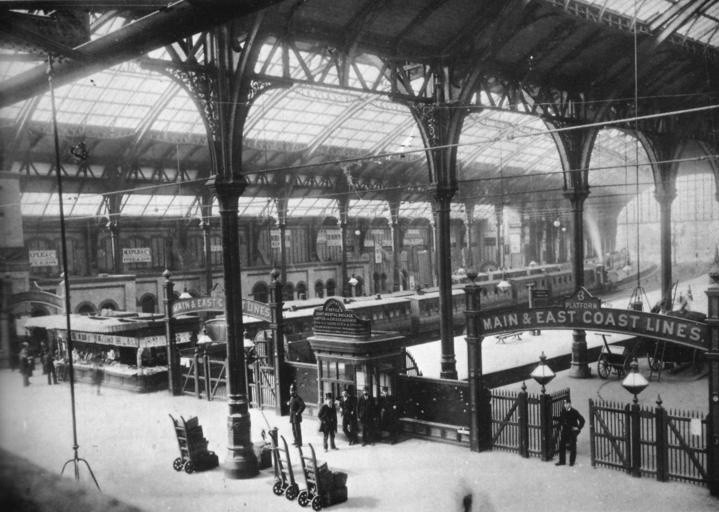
[564,399,571,404]
[325,393,333,400]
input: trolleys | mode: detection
[169,413,218,473]
[597,332,636,378]
[267,430,307,500]
[295,442,347,510]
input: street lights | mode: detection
[530,349,557,462]
[623,363,648,479]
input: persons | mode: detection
[339,389,361,445]
[374,386,400,445]
[38,339,60,386]
[17,340,34,387]
[553,398,586,466]
[285,383,306,446]
[316,393,339,451]
[91,357,105,394]
[356,386,379,448]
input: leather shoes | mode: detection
[292,436,398,453]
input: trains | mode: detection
[202,259,612,346]
